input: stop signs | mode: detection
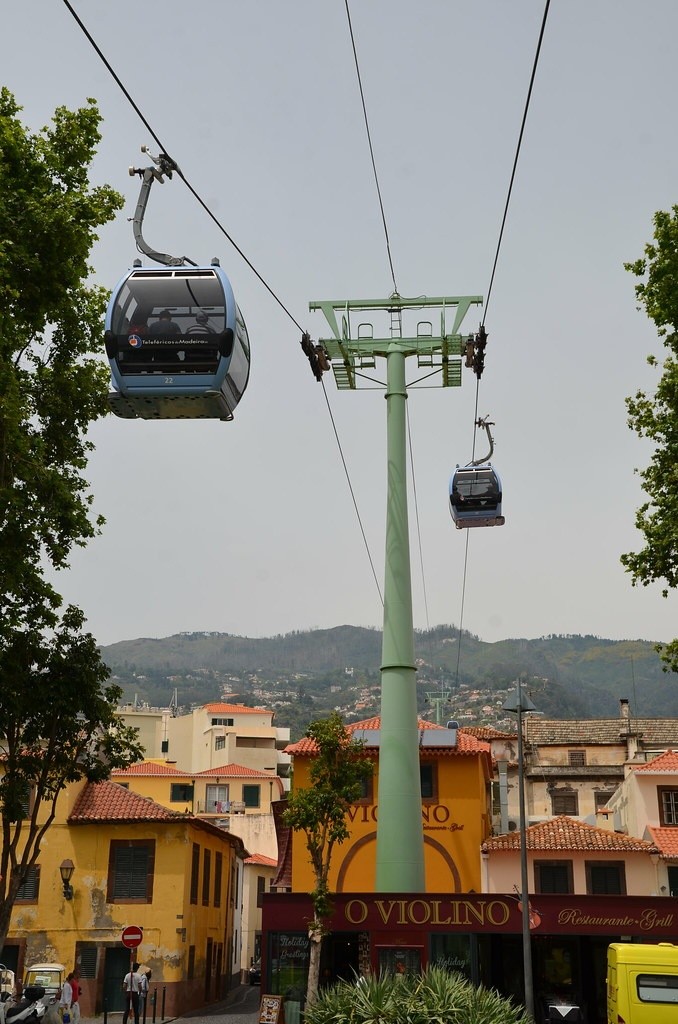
[122,925,143,948]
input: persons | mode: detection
[149,309,183,341]
[129,313,148,337]
[186,309,217,336]
[249,964,257,985]
[59,968,82,1023]
[123,963,153,1024]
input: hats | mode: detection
[196,310,210,321]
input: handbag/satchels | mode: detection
[55,990,62,1000]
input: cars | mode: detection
[272,959,283,975]
[249,958,262,986]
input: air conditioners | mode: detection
[494,816,520,834]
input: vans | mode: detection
[22,964,65,1008]
[606,942,678,1024]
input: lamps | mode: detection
[59,859,75,900]
